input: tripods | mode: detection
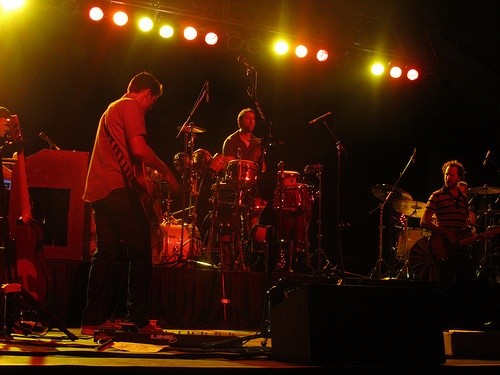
[158,90,220,273]
[294,169,343,278]
[368,191,414,281]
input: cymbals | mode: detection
[392,199,427,219]
[476,209,500,214]
[180,125,207,133]
[467,185,500,195]
[371,182,414,204]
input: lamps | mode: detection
[60,0,420,82]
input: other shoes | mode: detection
[138,320,166,334]
[80,320,123,335]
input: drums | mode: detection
[227,160,258,191]
[173,152,189,169]
[408,235,442,280]
[278,170,301,206]
[153,217,201,266]
[395,227,432,260]
[297,183,311,210]
[192,148,212,175]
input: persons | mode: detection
[84,71,181,335]
[419,160,495,332]
[222,107,269,239]
[0,106,14,217]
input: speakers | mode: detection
[271,283,446,366]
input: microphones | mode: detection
[483,149,490,165]
[37,130,61,150]
[206,80,210,104]
[412,148,416,164]
[309,112,332,125]
[243,60,257,72]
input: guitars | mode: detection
[428,226,499,263]
[4,112,54,308]
[139,169,162,226]
